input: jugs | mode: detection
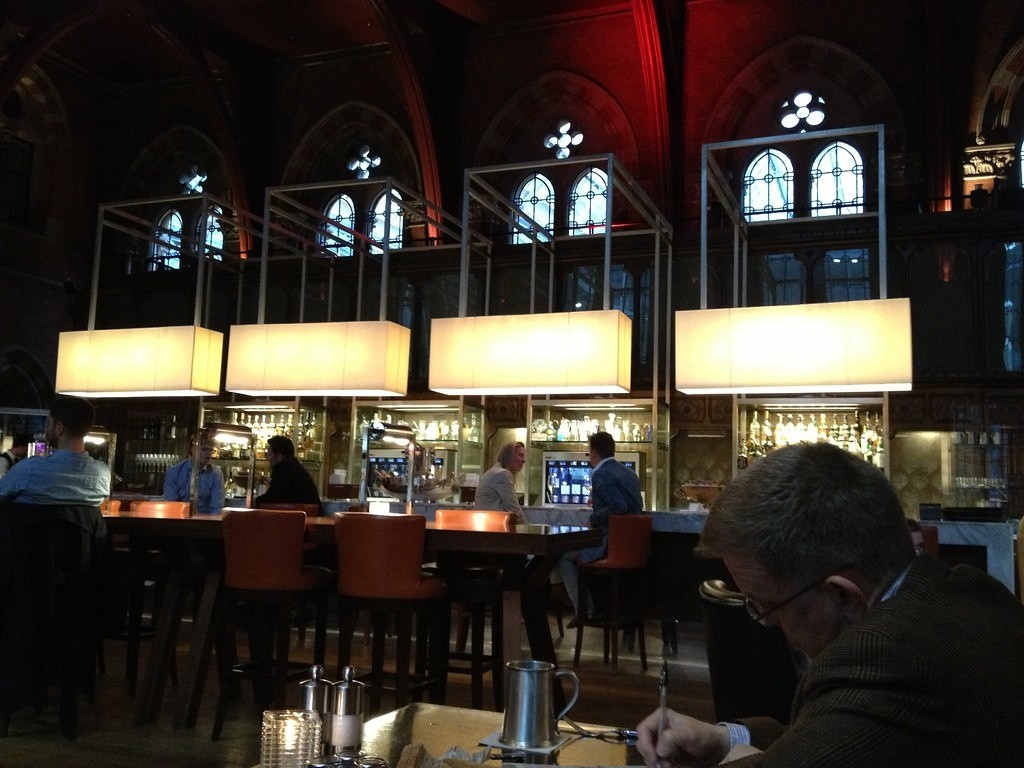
[501,660,582,768]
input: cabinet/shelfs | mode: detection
[197,397,331,501]
[523,398,672,507]
[345,395,485,501]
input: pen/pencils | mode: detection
[657,663,669,743]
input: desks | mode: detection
[98,498,602,730]
[251,701,643,768]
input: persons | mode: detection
[907,517,926,552]
[558,431,643,630]
[164,435,224,514]
[0,436,28,480]
[635,441,1024,768]
[474,441,530,524]
[0,396,111,507]
[253,435,323,517]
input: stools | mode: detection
[571,513,653,674]
[1,505,117,739]
[137,508,508,740]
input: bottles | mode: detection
[298,662,388,768]
[737,409,886,474]
[134,453,179,473]
[233,412,316,459]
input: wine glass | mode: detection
[622,420,631,440]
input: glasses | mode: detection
[745,573,840,629]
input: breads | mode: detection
[395,742,452,768]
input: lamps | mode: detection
[55,326,225,399]
[427,309,633,395]
[674,297,915,396]
[227,320,410,397]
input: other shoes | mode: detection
[567,608,593,630]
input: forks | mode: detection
[563,714,638,739]
